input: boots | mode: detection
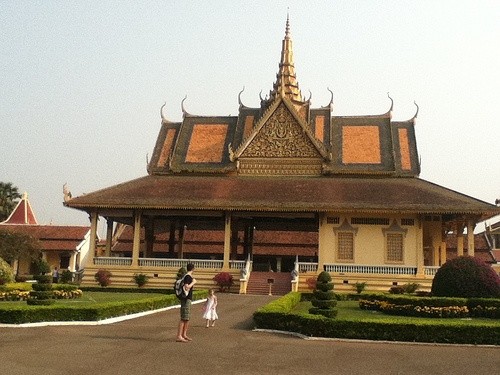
[211,321,215,326]
[206,319,209,327]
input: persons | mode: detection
[313,252,318,270]
[52,267,58,283]
[176,263,198,342]
[202,289,218,328]
[68,265,79,272]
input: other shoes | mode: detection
[182,335,192,340]
[176,336,188,342]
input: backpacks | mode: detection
[173,274,193,301]
